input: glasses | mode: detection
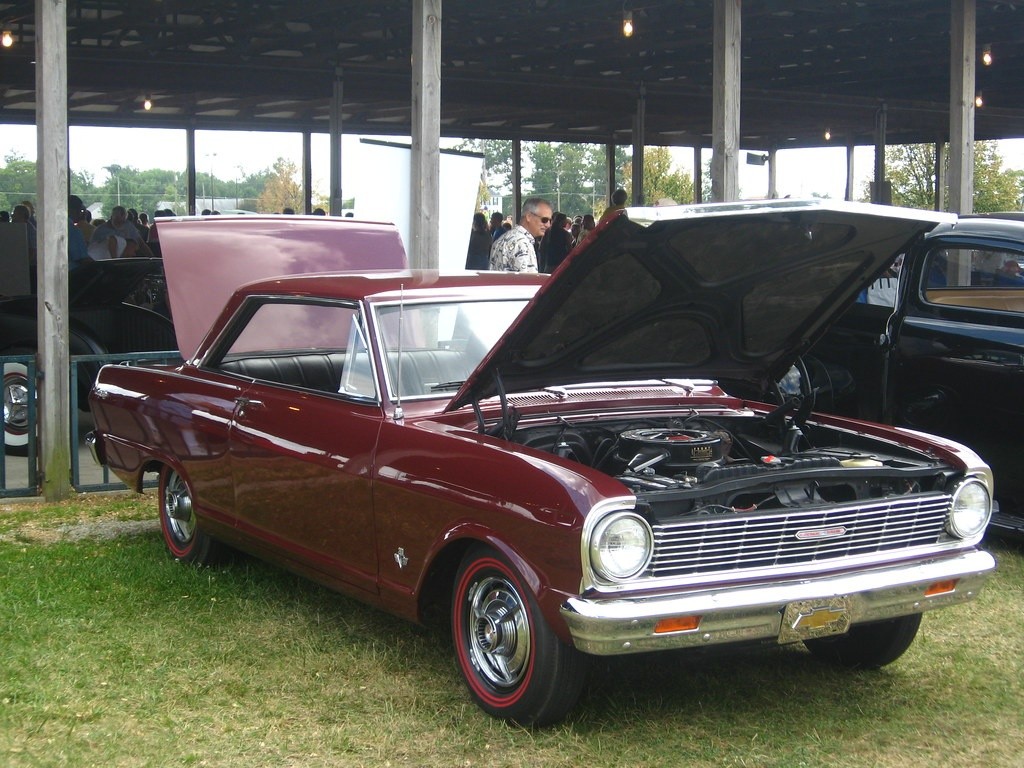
[531,212,552,225]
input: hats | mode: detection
[20,201,35,215]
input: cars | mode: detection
[1,217,180,460]
[84,197,997,732]
[785,213,1024,546]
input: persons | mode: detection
[0,194,222,308]
[283,207,354,217]
[995,260,1024,287]
[465,187,628,274]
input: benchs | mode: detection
[216,350,491,398]
[925,289,1024,313]
